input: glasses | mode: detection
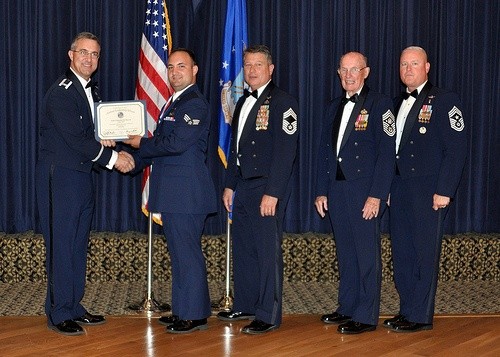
[339,67,366,75]
[71,49,100,59]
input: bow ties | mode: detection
[85,80,98,88]
[342,93,359,106]
[403,89,418,100]
[243,88,258,99]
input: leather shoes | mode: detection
[337,320,377,334]
[241,319,280,334]
[168,317,209,334]
[321,311,352,324]
[384,314,406,327]
[217,310,256,320]
[47,319,85,335]
[392,320,433,332]
[73,312,106,325]
[159,315,179,325]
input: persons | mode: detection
[313,52,396,335]
[42,32,135,336]
[216,44,300,335]
[115,51,218,334]
[386,45,468,333]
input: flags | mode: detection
[217,0,250,224]
[133,0,172,227]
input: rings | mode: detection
[372,211,376,214]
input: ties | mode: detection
[159,96,173,122]
